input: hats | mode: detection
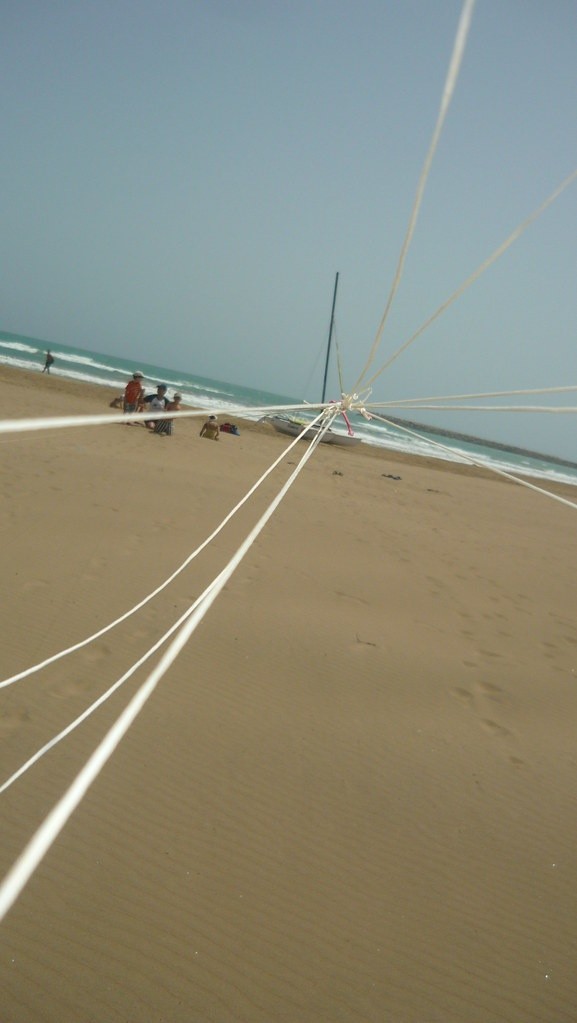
[174,393,182,399]
[133,371,146,378]
[157,384,167,391]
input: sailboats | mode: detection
[265,271,363,447]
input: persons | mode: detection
[139,384,168,428]
[123,371,144,425]
[199,415,220,440]
[42,349,53,374]
[155,393,182,435]
